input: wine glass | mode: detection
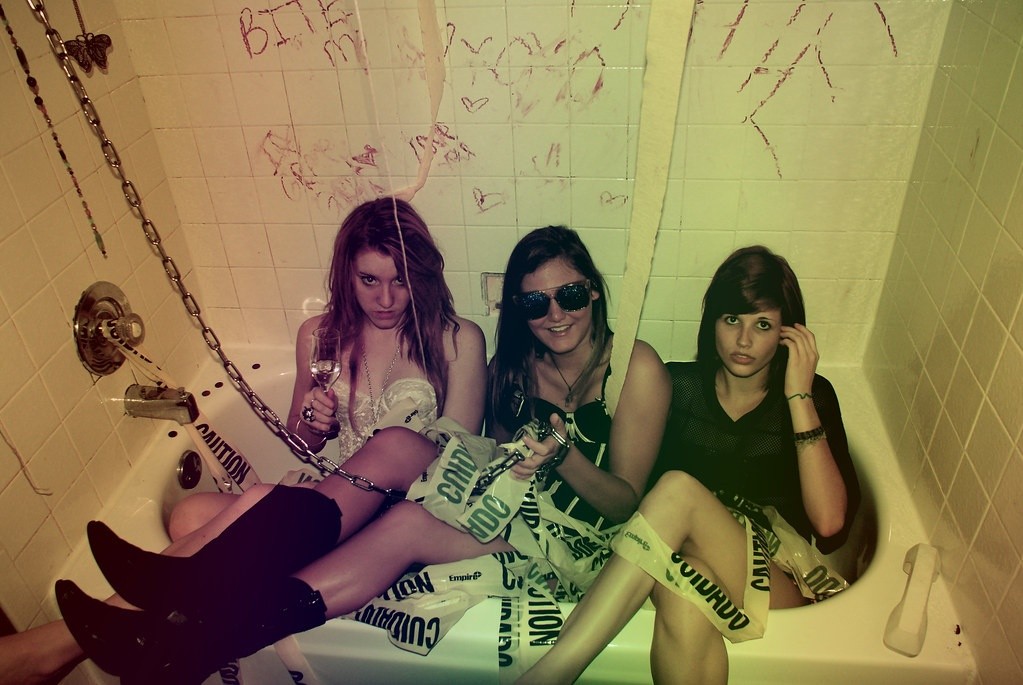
[304,326,342,435]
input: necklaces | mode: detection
[543,337,598,408]
[357,328,404,427]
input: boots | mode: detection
[88,484,343,611]
[56,579,326,685]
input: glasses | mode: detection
[512,279,590,320]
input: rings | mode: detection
[301,406,316,423]
[310,398,318,409]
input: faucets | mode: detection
[122,383,200,427]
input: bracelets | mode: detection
[293,418,327,447]
[786,392,813,401]
[793,424,826,446]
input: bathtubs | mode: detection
[57,362,974,684]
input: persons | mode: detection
[53,226,673,685]
[510,244,860,685]
[0,196,487,685]
